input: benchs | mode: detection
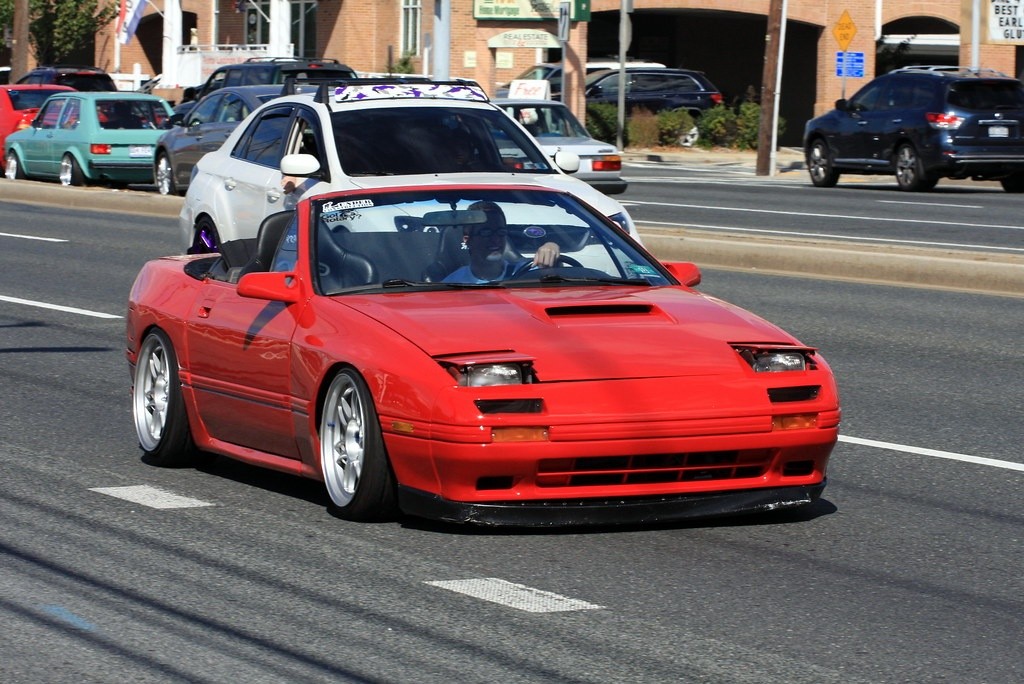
[207,232,445,285]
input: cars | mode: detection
[154,83,341,196]
[3,92,174,190]
[491,79,628,198]
[0,83,78,176]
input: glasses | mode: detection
[468,227,507,237]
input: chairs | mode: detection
[237,210,377,294]
[422,224,525,284]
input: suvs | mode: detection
[178,77,649,279]
[181,58,357,93]
[803,62,1024,193]
[14,64,117,94]
[498,56,722,148]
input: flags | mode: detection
[114,0,150,45]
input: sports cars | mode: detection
[127,185,841,529]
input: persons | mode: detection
[437,201,561,284]
